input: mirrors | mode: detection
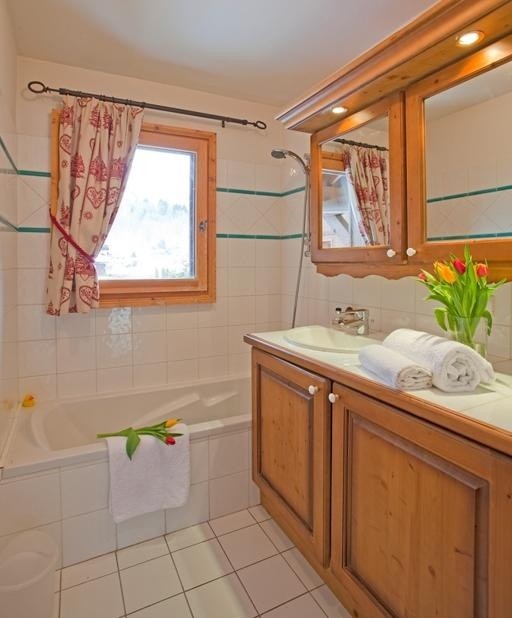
[318,114,389,248]
[423,60,512,240]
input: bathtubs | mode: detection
[1,372,260,571]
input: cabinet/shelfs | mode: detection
[243,329,512,618]
[311,34,512,264]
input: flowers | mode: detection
[416,241,507,336]
[97,418,185,460]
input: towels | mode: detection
[359,344,432,391]
[105,423,191,523]
[382,328,496,392]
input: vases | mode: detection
[454,318,487,359]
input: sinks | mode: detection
[285,324,382,351]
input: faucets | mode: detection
[337,309,370,335]
